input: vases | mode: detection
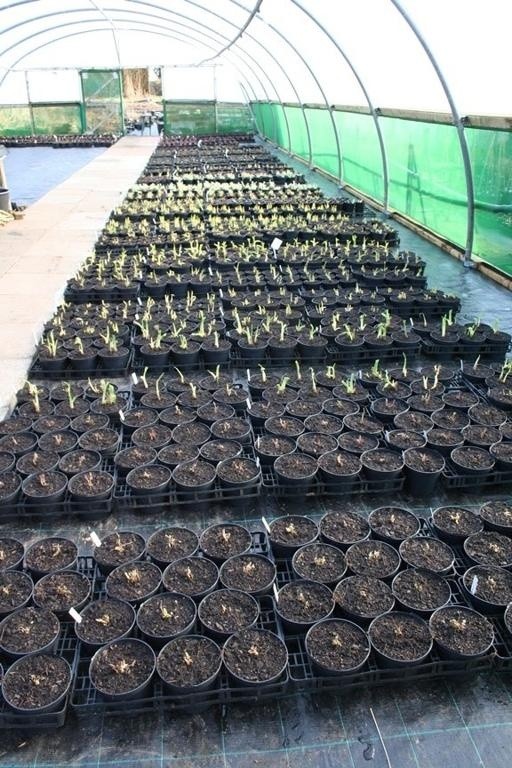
[0,133,123,148]
[358,198,511,361]
[35,190,131,377]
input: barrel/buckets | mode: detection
[0,188,12,211]
